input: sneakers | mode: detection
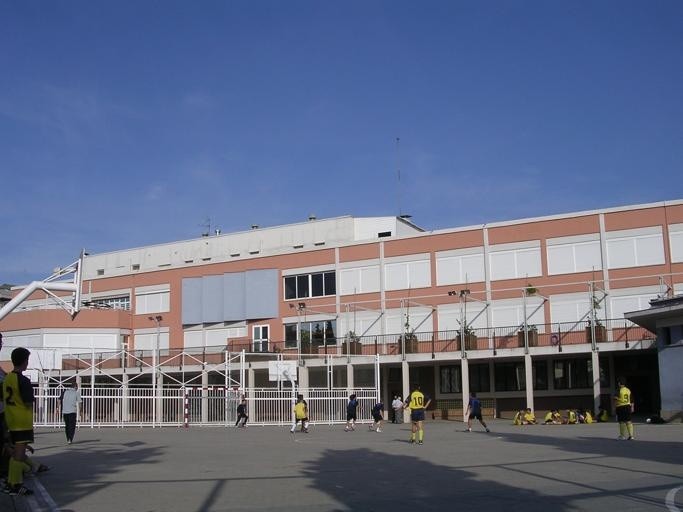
[616,435,626,441]
[290,428,307,433]
[464,427,471,432]
[24,464,55,479]
[627,436,635,440]
[411,440,423,445]
[369,424,382,433]
[486,428,490,432]
[234,424,248,428]
[344,424,356,432]
[3,483,34,497]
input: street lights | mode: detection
[447,289,471,358]
[289,302,305,365]
[148,316,162,377]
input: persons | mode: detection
[234,400,248,427]
[290,395,309,433]
[392,396,403,424]
[513,406,608,425]
[403,382,432,445]
[62,382,82,444]
[611,377,635,441]
[0,333,48,496]
[465,393,490,432]
[344,395,359,432]
[371,400,384,432]
